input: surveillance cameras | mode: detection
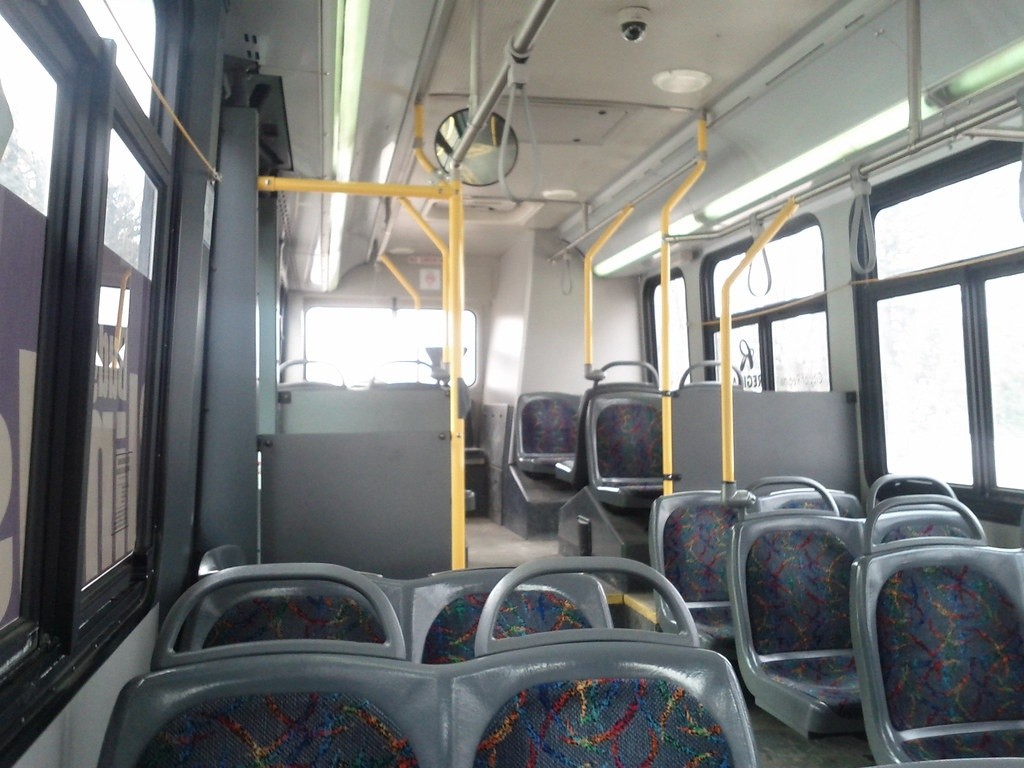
[615,7,651,42]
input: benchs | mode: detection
[95,360,1024,768]
